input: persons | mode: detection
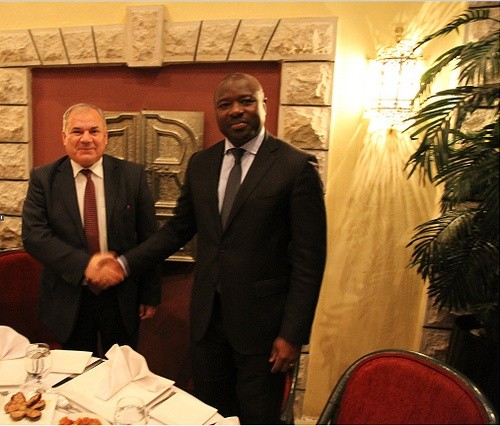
[21,103,163,361]
[96,72,328,426]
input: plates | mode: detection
[55,412,112,426]
[0,390,61,426]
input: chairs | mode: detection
[314,348,500,425]
[0,247,44,338]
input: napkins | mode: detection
[90,344,151,401]
[0,325,32,359]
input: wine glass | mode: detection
[26,343,51,394]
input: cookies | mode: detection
[4,392,45,420]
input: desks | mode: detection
[0,351,242,425]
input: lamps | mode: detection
[368,26,430,131]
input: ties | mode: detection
[217,148,246,293]
[79,169,100,256]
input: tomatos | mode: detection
[57,416,102,425]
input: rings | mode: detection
[289,364,295,366]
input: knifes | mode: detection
[51,359,103,388]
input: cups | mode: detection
[115,395,148,426]
